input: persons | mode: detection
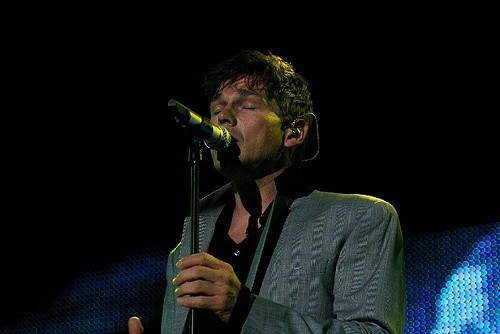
[128,51,407,334]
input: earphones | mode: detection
[290,124,299,137]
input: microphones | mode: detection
[167,99,233,152]
